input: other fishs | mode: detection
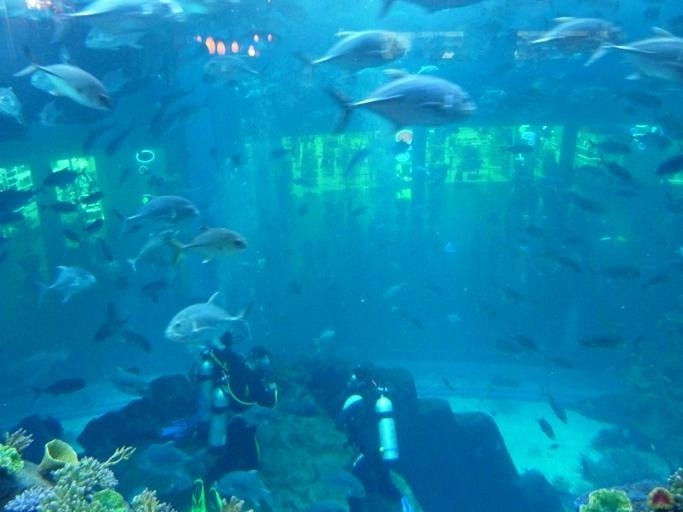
[1,0,683,512]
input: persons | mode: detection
[204,346,278,488]
[335,365,414,512]
